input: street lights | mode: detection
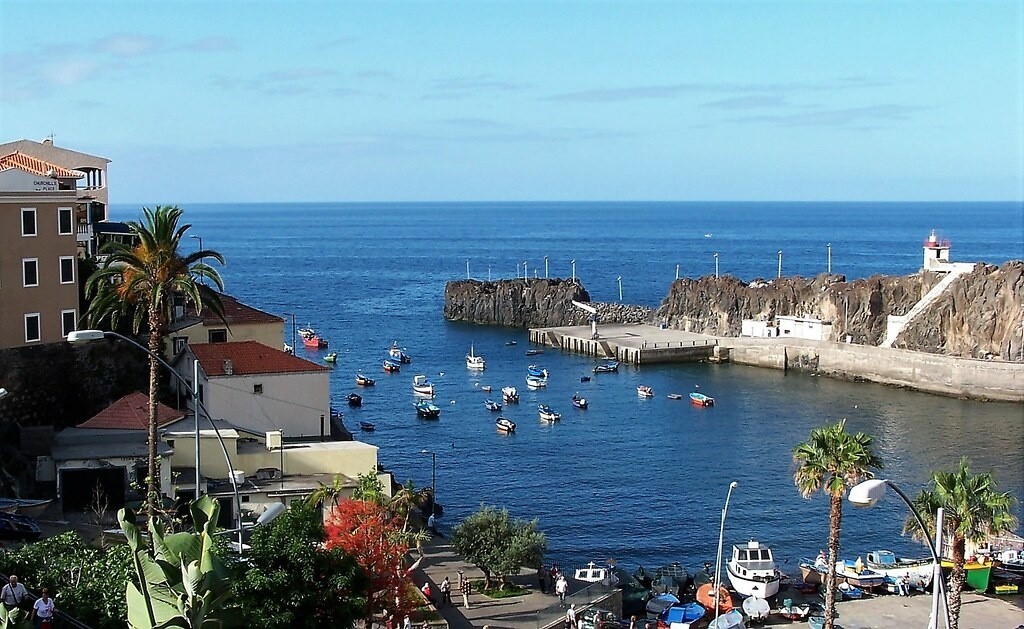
[282,312,296,356]
[848,477,952,629]
[67,330,242,560]
[422,450,436,514]
[189,234,203,284]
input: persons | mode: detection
[0,575,28,629]
[30,587,55,629]
[540,565,568,609]
[629,615,639,629]
[421,582,431,597]
[885,573,889,578]
[703,561,716,574]
[461,576,471,610]
[902,571,911,596]
[592,610,602,629]
[440,576,453,604]
[564,604,585,629]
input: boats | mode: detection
[689,392,714,407]
[411,375,434,395]
[797,550,992,600]
[572,394,588,408]
[725,538,781,602]
[537,404,561,421]
[994,579,1020,595]
[667,393,682,399]
[389,341,410,364]
[0,496,54,544]
[837,582,862,600]
[481,385,492,391]
[637,385,652,396]
[383,359,400,372]
[502,385,519,402]
[413,398,441,419]
[323,352,337,362]
[485,399,502,411]
[495,417,516,432]
[297,326,328,348]
[577,562,771,629]
[354,372,376,386]
[348,393,362,405]
[593,361,619,372]
[775,597,837,629]
[818,583,842,601]
[359,421,375,430]
[525,365,550,387]
[465,345,486,368]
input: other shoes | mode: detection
[563,606,566,608]
[560,605,562,607]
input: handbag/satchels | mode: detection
[441,586,446,592]
[16,602,24,609]
